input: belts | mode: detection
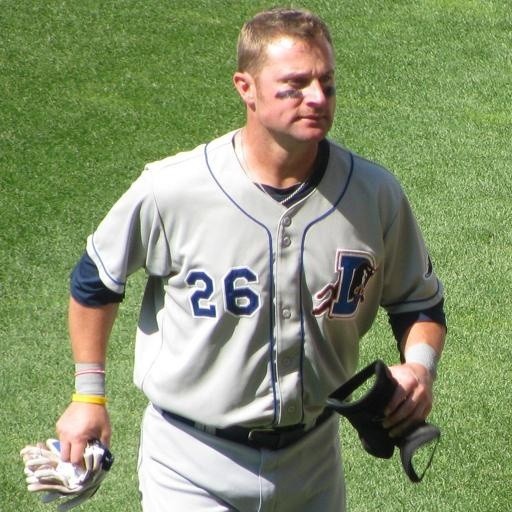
[162,407,333,449]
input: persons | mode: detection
[55,8,446,512]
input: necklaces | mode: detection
[241,129,319,204]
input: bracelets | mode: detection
[404,342,439,371]
[72,363,106,404]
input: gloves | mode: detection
[20,439,114,512]
